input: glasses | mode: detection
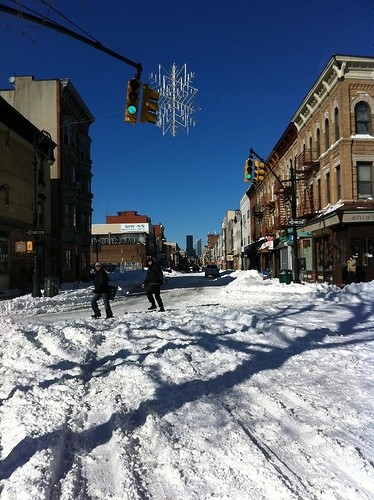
[96,266,99,267]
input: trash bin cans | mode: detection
[44,275,59,296]
[279,269,292,283]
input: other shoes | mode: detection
[158,309,165,312]
[105,316,113,319]
[148,305,156,309]
[91,314,101,317]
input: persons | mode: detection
[90,262,113,319]
[347,255,357,283]
[142,258,166,312]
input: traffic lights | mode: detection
[140,84,159,124]
[124,79,140,124]
[254,160,265,182]
[244,158,253,182]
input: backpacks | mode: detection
[151,264,163,283]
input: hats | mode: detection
[146,258,152,261]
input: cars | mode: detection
[204,265,220,277]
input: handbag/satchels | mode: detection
[108,285,117,299]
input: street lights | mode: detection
[234,209,244,271]
[31,129,56,298]
